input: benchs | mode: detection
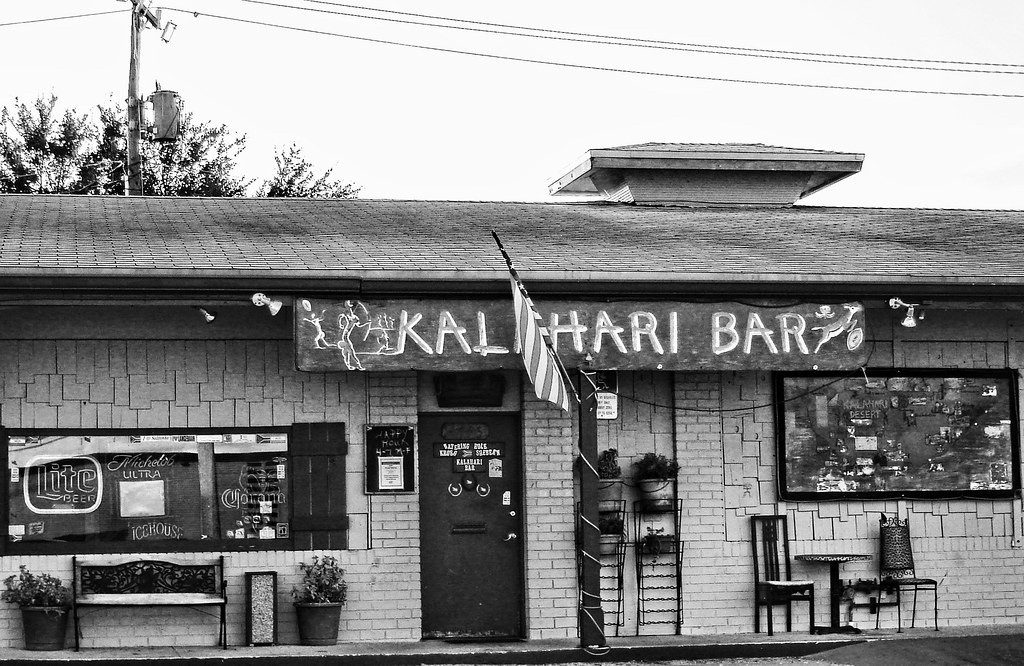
[68,554,228,652]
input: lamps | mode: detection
[251,292,284,315]
[198,310,215,319]
[890,297,921,328]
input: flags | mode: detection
[510,276,571,417]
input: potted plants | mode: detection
[291,555,348,644]
[632,452,680,509]
[645,526,674,553]
[600,517,624,552]
[0,563,69,651]
[600,447,624,508]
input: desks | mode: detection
[794,553,873,635]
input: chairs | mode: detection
[751,514,815,637]
[873,517,939,633]
